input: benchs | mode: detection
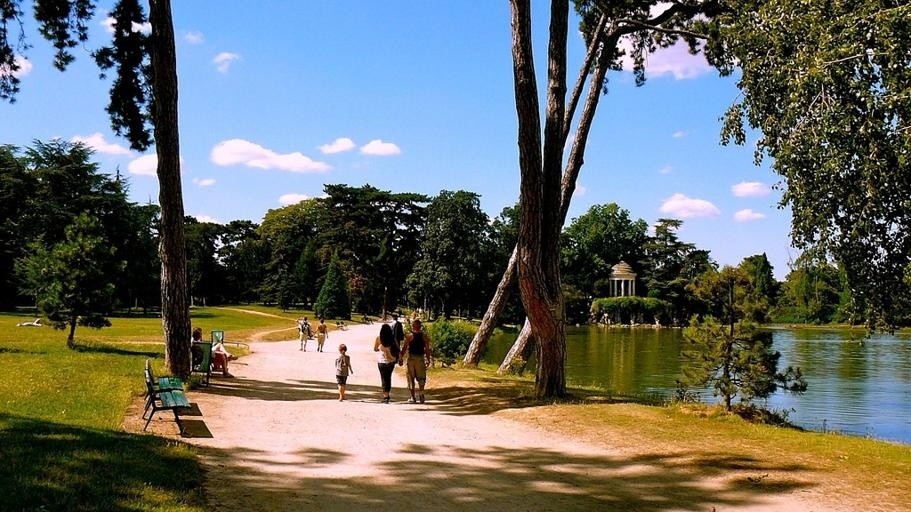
[142,370,191,436]
[144,359,183,409]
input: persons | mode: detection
[335,319,346,330]
[373,323,402,403]
[588,310,644,325]
[403,318,412,346]
[297,316,311,352]
[190,326,238,361]
[192,330,234,378]
[390,308,417,327]
[400,320,430,403]
[390,317,404,364]
[334,343,354,401]
[364,314,372,324]
[312,318,329,352]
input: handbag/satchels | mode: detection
[390,344,399,357]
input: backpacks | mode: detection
[301,322,310,334]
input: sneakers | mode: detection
[317,347,323,352]
[382,396,391,404]
[300,348,306,352]
[407,394,425,404]
